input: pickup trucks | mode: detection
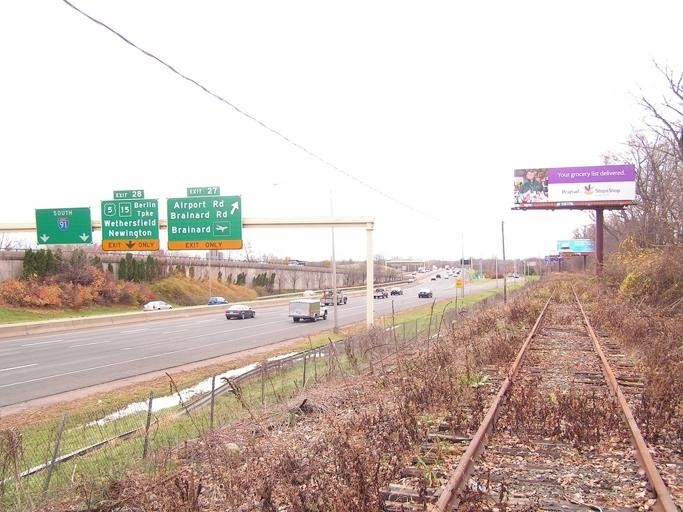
[373,287,388,299]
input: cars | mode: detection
[430,264,462,281]
[390,288,403,296]
[411,266,430,276]
[207,297,230,305]
[418,289,432,298]
[142,300,173,312]
[507,272,519,279]
[226,304,256,320]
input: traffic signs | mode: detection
[166,196,245,251]
[185,186,220,197]
[100,199,160,253]
[34,208,92,244]
[113,188,144,201]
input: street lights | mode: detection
[273,175,341,335]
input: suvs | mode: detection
[320,289,347,306]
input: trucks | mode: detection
[285,260,306,267]
[288,299,328,322]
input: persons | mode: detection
[519,170,545,195]
